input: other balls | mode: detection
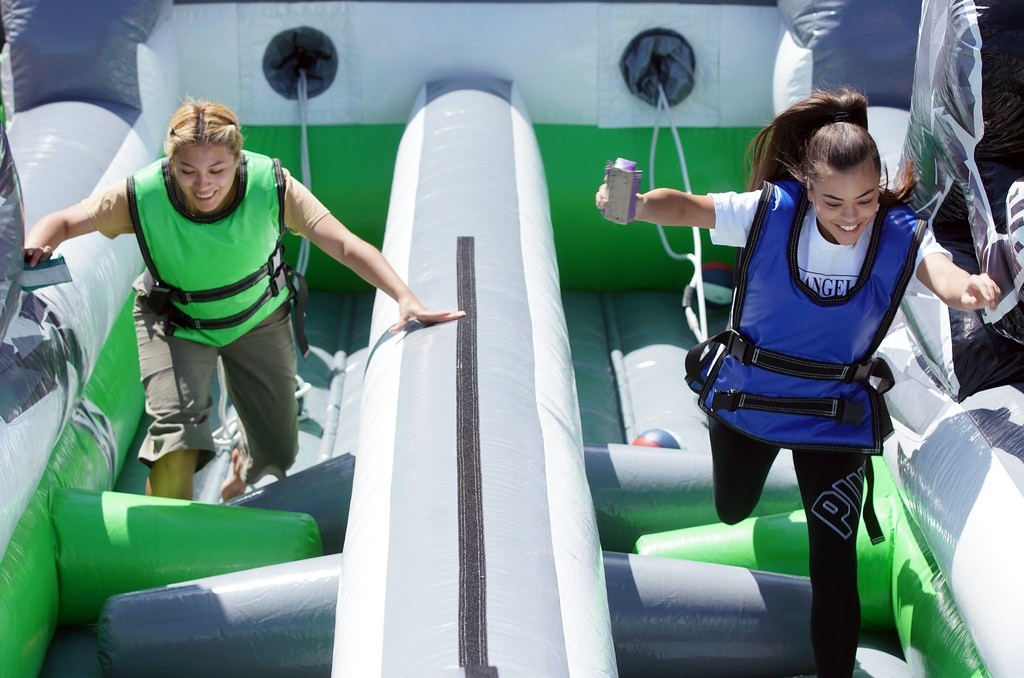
[696,263,735,310]
[632,427,686,451]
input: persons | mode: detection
[25,101,467,500]
[596,89,1002,678]
